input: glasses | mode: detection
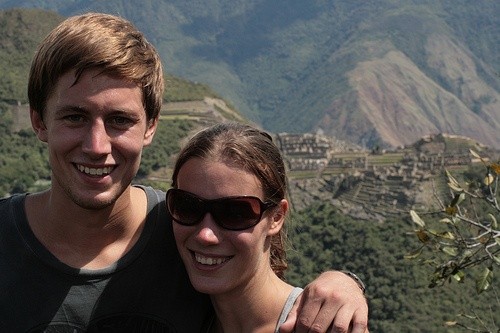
[165,189,275,231]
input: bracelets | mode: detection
[340,270,366,294]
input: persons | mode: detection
[1,12,369,332]
